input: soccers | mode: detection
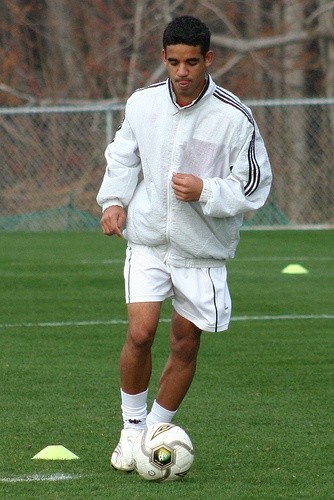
[131,422,195,483]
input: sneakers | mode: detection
[110,430,141,470]
